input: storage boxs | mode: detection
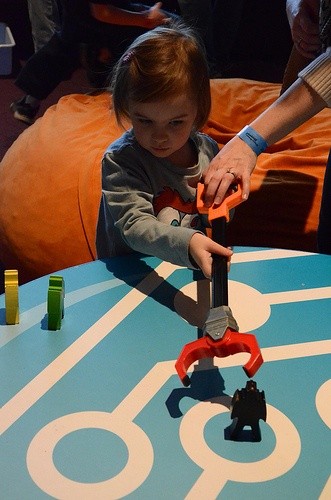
[0,23,16,75]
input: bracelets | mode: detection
[238,125,269,157]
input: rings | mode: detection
[297,39,303,46]
[226,168,239,180]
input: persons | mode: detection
[9,0,168,126]
[95,26,233,280]
[202,46,331,255]
[280,0,331,96]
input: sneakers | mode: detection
[10,95,41,125]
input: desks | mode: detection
[0,246,331,500]
